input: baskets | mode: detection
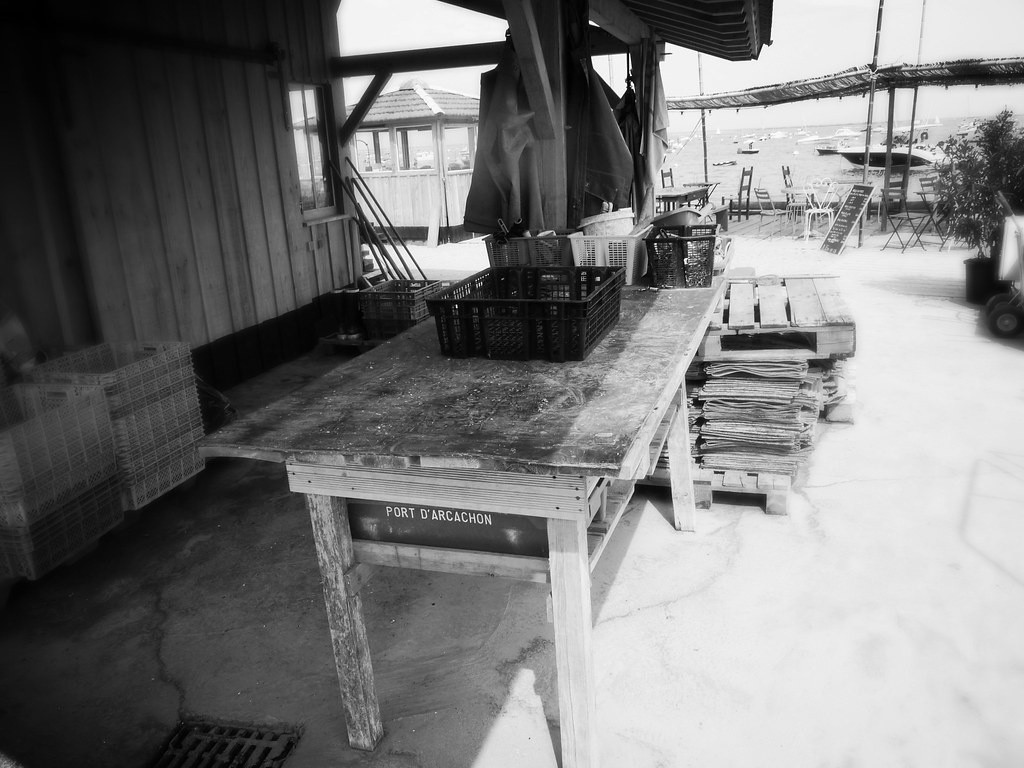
[425,267,626,361]
[0,338,205,582]
[358,279,443,321]
[363,315,431,340]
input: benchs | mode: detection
[696,205,729,231]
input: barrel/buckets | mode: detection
[577,210,634,236]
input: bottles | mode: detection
[331,289,360,320]
[825,181,830,185]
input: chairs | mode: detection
[661,166,950,253]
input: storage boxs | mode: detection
[0,339,206,582]
[344,224,718,363]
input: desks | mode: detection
[196,287,721,768]
[781,185,843,238]
[656,187,709,213]
[816,180,873,218]
[684,182,720,209]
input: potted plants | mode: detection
[930,111,1024,304]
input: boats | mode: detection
[782,145,964,207]
[737,142,759,154]
[713,161,737,166]
[817,148,839,154]
[759,115,944,144]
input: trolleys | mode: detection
[984,191,1024,337]
